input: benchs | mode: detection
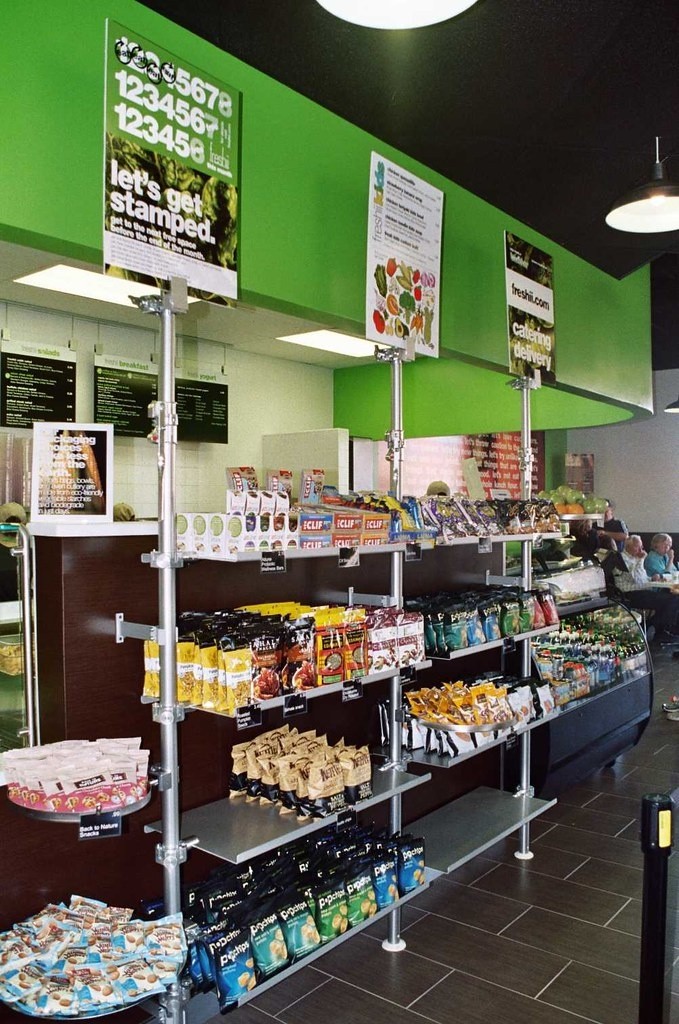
[609,574,658,640]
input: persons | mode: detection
[569,499,629,566]
[426,481,450,497]
[611,534,677,643]
[644,533,678,581]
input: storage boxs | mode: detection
[176,467,389,555]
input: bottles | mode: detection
[531,615,647,704]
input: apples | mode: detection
[540,486,608,514]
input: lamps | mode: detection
[605,137,679,233]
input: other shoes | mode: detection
[653,632,674,643]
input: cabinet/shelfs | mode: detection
[1,530,565,1022]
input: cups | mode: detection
[663,574,672,581]
[671,571,679,584]
